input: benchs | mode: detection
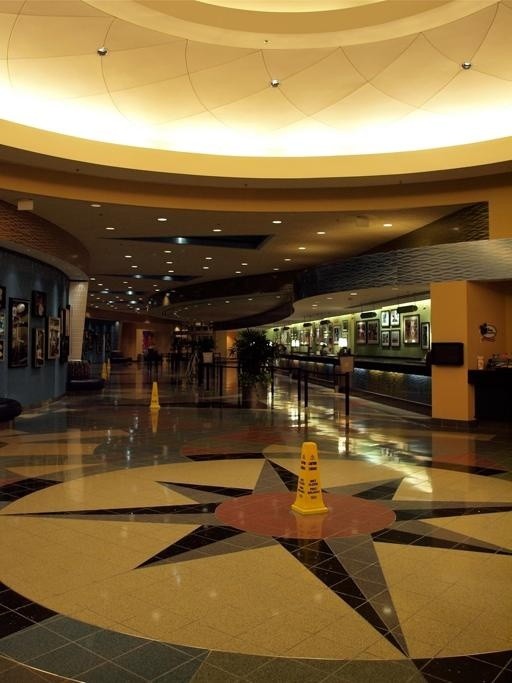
[67,360,105,392]
[0,398,22,429]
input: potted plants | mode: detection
[229,326,291,409]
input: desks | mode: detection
[468,369,512,420]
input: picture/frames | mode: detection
[355,309,430,351]
[333,320,348,345]
[0,285,66,369]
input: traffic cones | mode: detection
[107,368,110,378]
[101,362,107,379]
[290,510,329,572]
[149,381,162,410]
[150,408,160,433]
[291,440,328,515]
[108,358,111,368]
